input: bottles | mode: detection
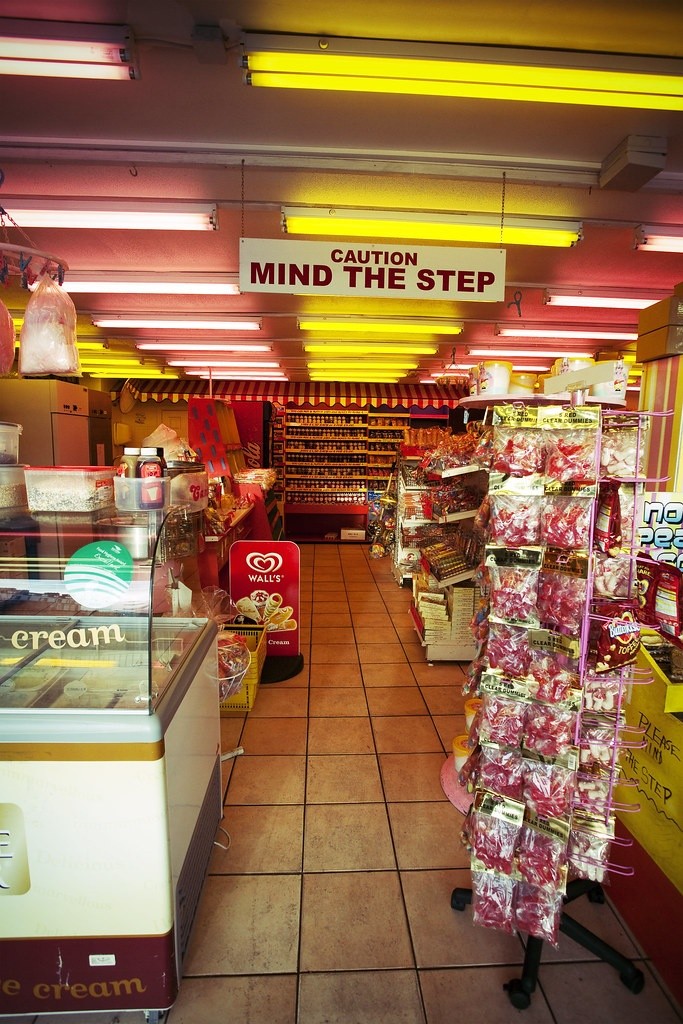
[283,414,398,506]
[215,483,234,513]
[154,447,166,468]
[137,448,162,478]
[120,447,140,479]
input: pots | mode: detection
[94,517,166,559]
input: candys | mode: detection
[458,423,648,946]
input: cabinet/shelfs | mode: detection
[394,453,436,587]
[285,409,367,542]
[413,443,488,659]
[368,412,410,543]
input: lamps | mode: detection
[0,16,143,81]
[279,206,584,248]
[0,200,218,233]
[237,32,683,113]
[633,223,683,254]
[28,269,661,384]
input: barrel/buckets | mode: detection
[468,366,478,396]
[538,357,632,400]
[477,361,512,396]
[0,463,30,508]
[509,374,537,396]
[0,421,23,464]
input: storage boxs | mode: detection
[24,466,115,513]
[219,626,268,713]
[417,587,492,645]
[114,475,171,512]
[635,295,683,361]
[615,643,682,893]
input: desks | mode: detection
[0,503,254,591]
